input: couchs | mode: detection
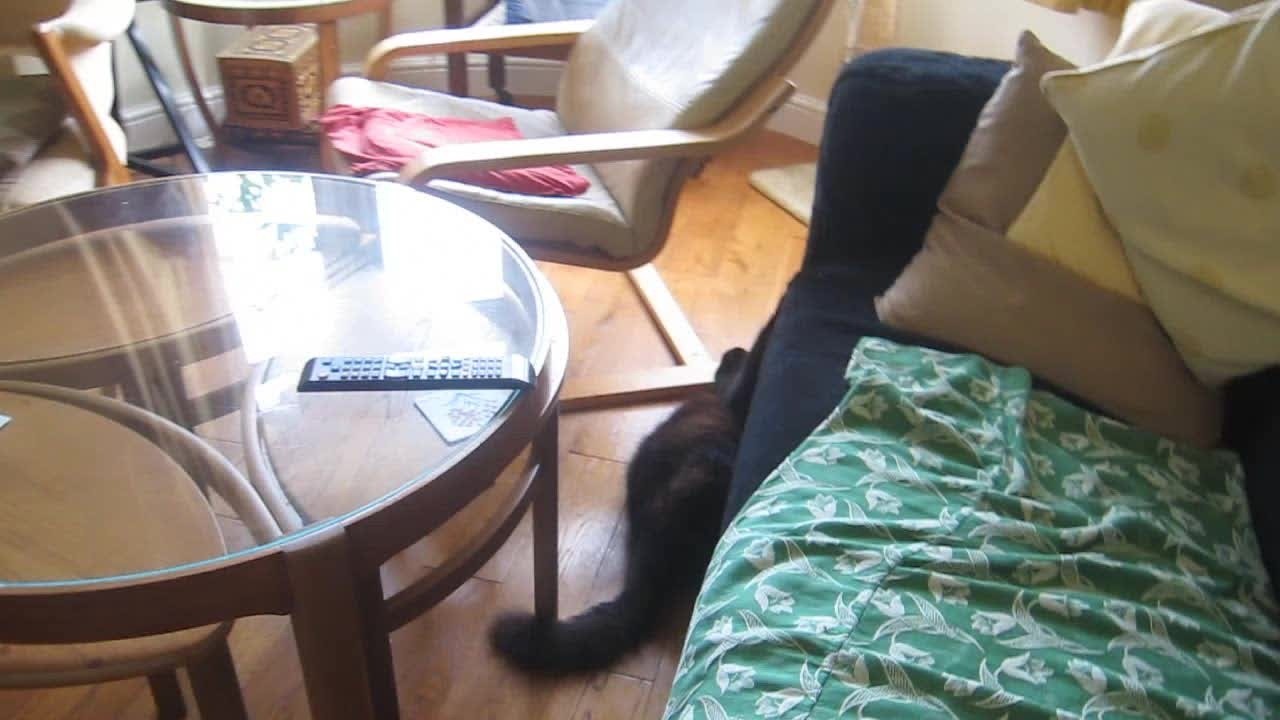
[657,43,1280,720]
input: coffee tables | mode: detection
[1,171,574,720]
[164,0,395,182]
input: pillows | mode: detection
[880,32,1229,450]
[1040,0,1279,390]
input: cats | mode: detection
[491,349,760,679]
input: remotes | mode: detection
[295,355,537,391]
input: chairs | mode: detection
[1,0,143,196]
[333,2,837,420]
[1,378,292,720]
